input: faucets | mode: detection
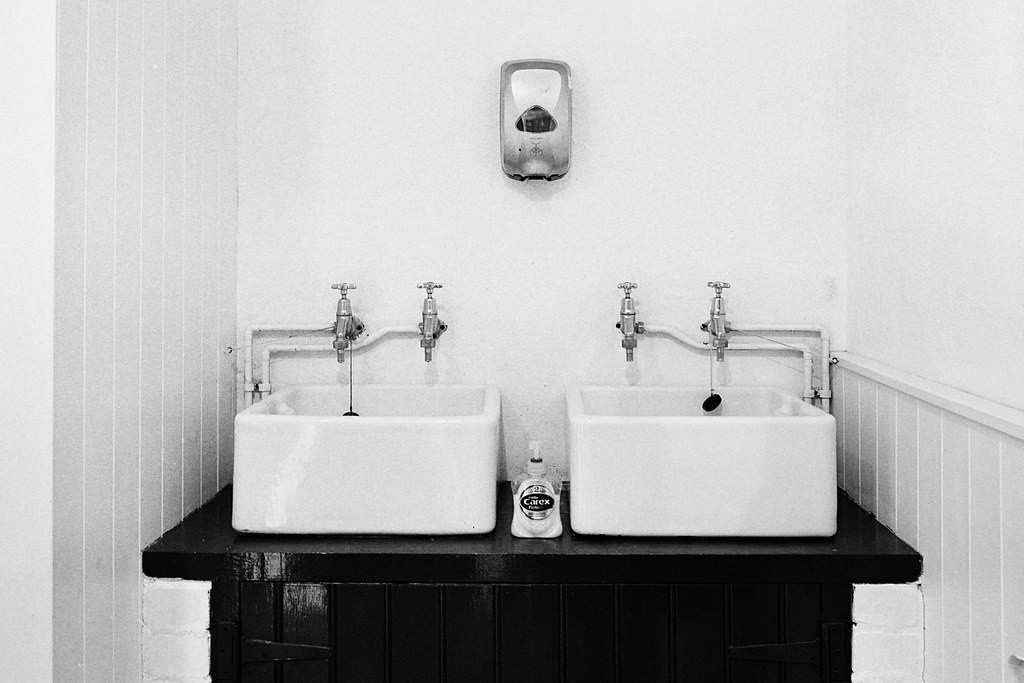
[417,282,444,363]
[617,282,639,361]
[707,282,731,363]
[332,282,363,364]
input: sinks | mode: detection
[233,381,501,536]
[564,384,839,538]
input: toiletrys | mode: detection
[508,441,564,539]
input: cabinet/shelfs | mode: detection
[211,581,854,683]
